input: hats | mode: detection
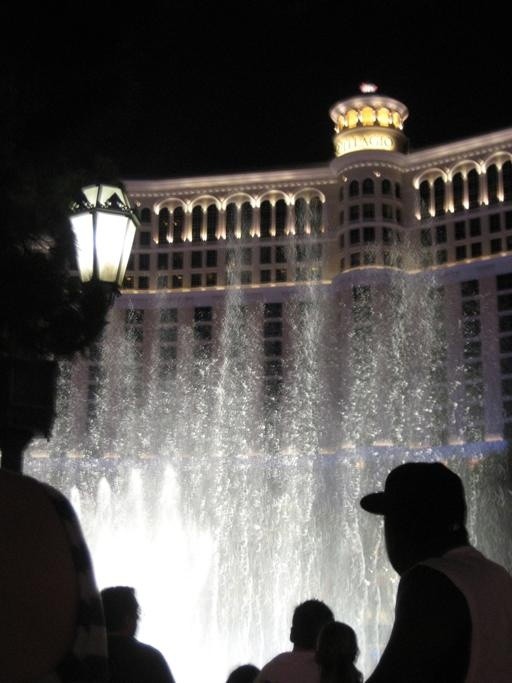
[361,462,467,518]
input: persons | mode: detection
[314,619,361,683]
[100,585,175,683]
[363,461,511,683]
[224,663,260,683]
[1,353,110,682]
[254,598,363,683]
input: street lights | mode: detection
[1,144,145,475]
